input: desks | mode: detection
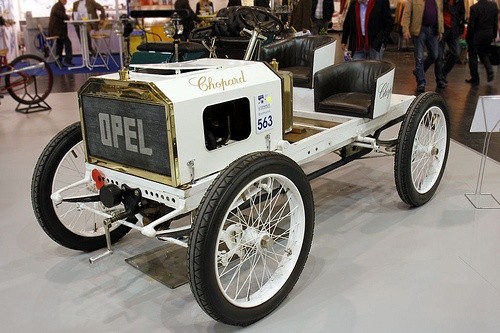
[266,11,292,22]
[64,20,106,71]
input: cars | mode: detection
[31,5,450,327]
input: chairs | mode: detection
[260,35,337,113]
[314,59,396,119]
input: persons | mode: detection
[254,0,271,8]
[401,0,445,95]
[434,0,465,93]
[340,0,394,63]
[72,0,106,58]
[464,0,500,86]
[288,0,312,32]
[0,16,16,62]
[174,0,205,43]
[311,0,335,36]
[196,0,214,15]
[48,0,77,67]
[227,0,242,7]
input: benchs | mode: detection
[189,6,270,47]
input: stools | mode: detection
[38,24,62,70]
[89,19,120,70]
